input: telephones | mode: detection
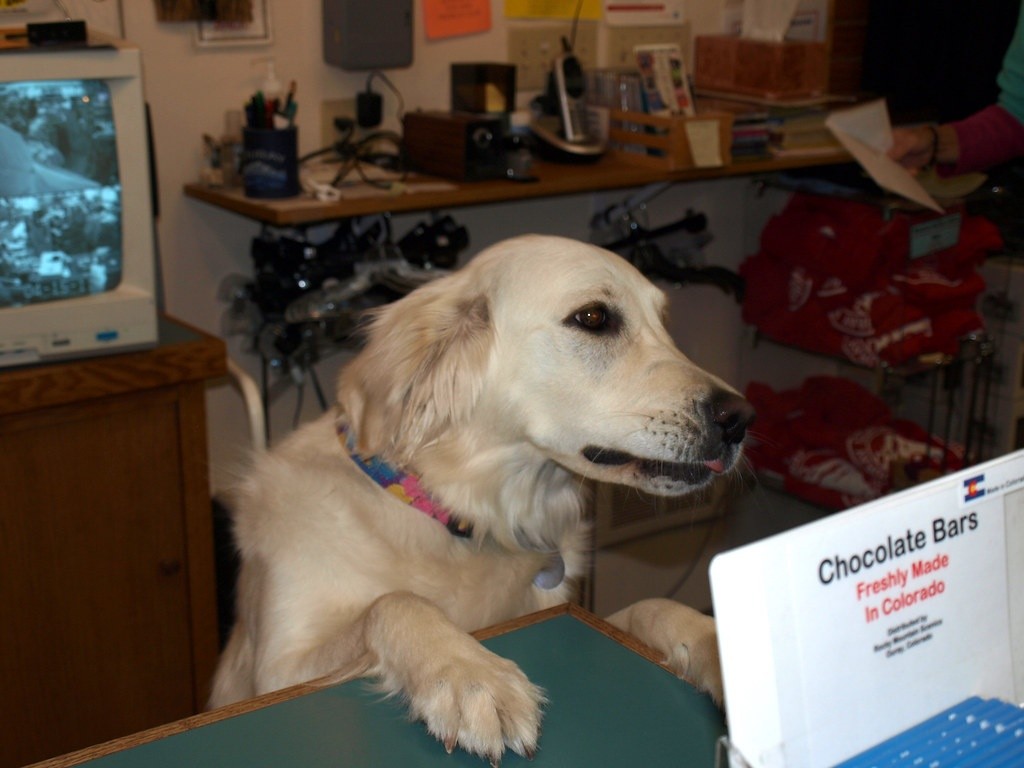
[530,35,604,161]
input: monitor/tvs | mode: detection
[0,45,160,370]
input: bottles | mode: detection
[220,110,246,189]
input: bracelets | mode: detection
[920,125,938,171]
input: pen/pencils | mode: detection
[243,79,299,126]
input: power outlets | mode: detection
[319,95,381,169]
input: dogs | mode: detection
[205,234,756,768]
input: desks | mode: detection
[182,144,854,446]
[22,600,729,768]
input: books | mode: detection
[695,98,771,159]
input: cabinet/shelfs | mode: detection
[0,312,229,768]
[733,171,1018,511]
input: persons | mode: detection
[887,0,1024,179]
